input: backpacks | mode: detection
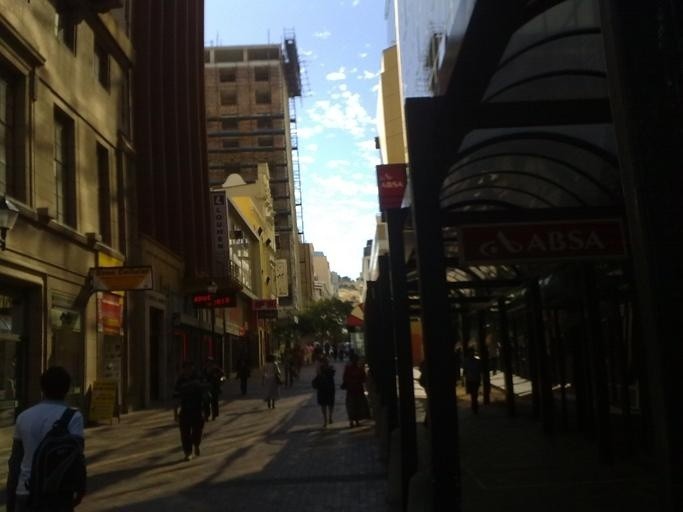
[24,405,86,509]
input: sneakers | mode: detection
[184,453,192,461]
[195,445,200,456]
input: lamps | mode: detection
[0,193,22,251]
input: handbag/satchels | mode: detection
[312,375,321,390]
[275,365,284,386]
[341,382,349,389]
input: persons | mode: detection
[259,353,281,410]
[310,357,335,429]
[202,355,226,422]
[172,372,209,462]
[235,359,249,396]
[4,364,86,511]
[282,338,354,388]
[340,352,370,428]
